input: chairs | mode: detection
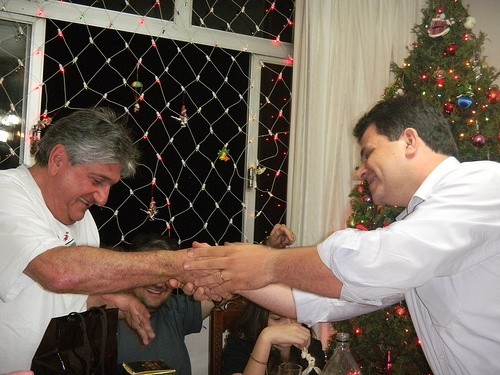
[209,295,247,375]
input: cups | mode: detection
[279,363,303,375]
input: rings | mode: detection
[220,269,225,282]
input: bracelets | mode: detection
[249,353,268,365]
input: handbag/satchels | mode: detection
[31,304,118,375]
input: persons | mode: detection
[0,107,232,375]
[170,99,500,375]
[115,222,296,375]
[223,302,325,375]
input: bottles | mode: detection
[319,332,363,375]
